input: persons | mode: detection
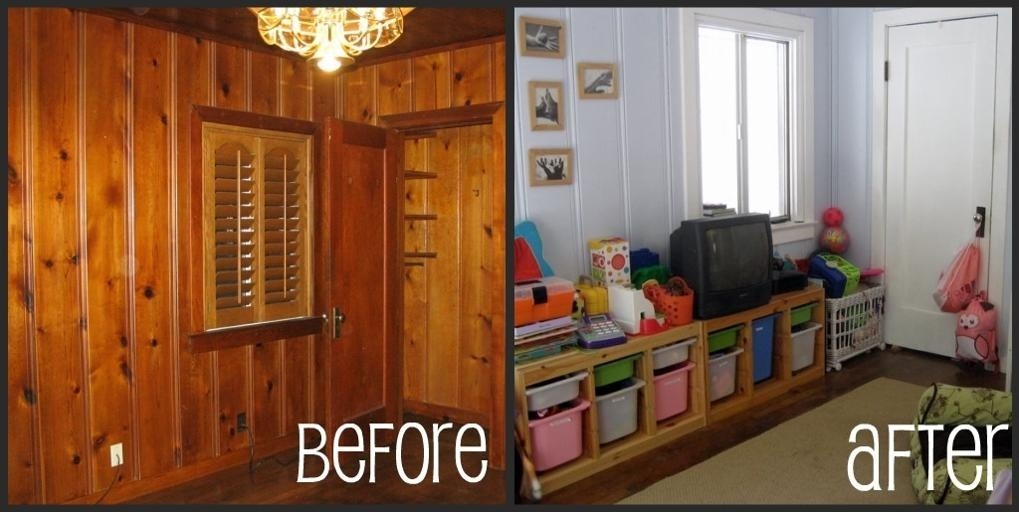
[526,26,560,52]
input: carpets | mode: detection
[613,376,930,505]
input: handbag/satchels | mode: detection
[934,244,979,312]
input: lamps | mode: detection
[247,5,419,78]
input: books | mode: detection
[514,314,581,366]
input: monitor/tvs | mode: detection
[670,214,774,320]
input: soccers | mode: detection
[820,228,849,253]
[823,207,843,227]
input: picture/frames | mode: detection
[527,147,573,187]
[519,15,566,58]
[528,81,565,131]
[577,62,620,98]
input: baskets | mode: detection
[645,278,694,326]
